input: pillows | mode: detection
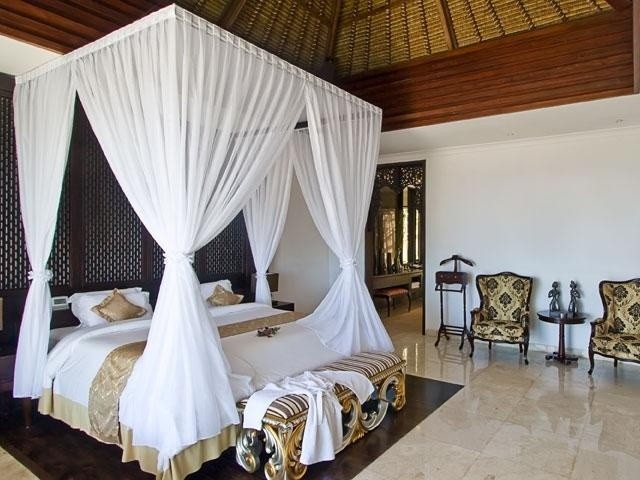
[63,277,247,330]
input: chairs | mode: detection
[461,271,640,373]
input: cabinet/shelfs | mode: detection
[364,268,422,317]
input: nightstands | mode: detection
[268,298,295,312]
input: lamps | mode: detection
[250,272,279,306]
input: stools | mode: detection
[373,286,412,318]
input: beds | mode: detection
[1,269,374,480]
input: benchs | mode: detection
[233,349,410,480]
